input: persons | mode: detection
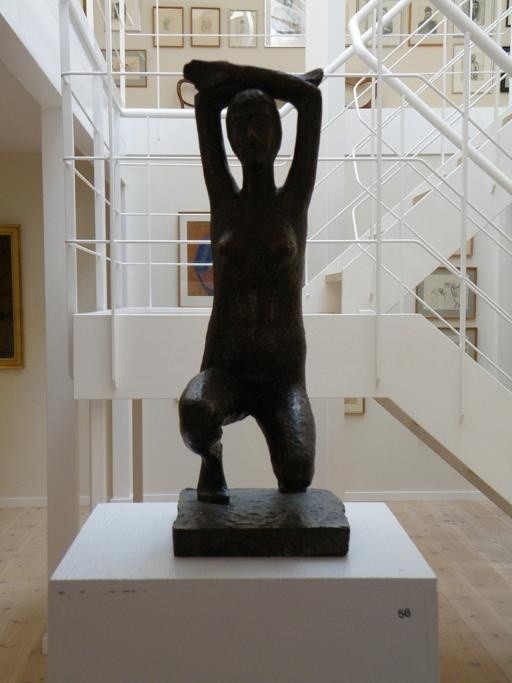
[177,54,326,504]
[416,5,437,34]
[272,0,302,34]
[470,54,479,81]
[376,6,393,34]
[467,0,481,24]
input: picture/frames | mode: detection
[153,0,305,47]
[0,224,24,369]
[177,211,213,307]
[416,237,478,361]
[452,43,512,94]
[344,398,365,415]
[101,0,147,87]
[346,0,512,48]
